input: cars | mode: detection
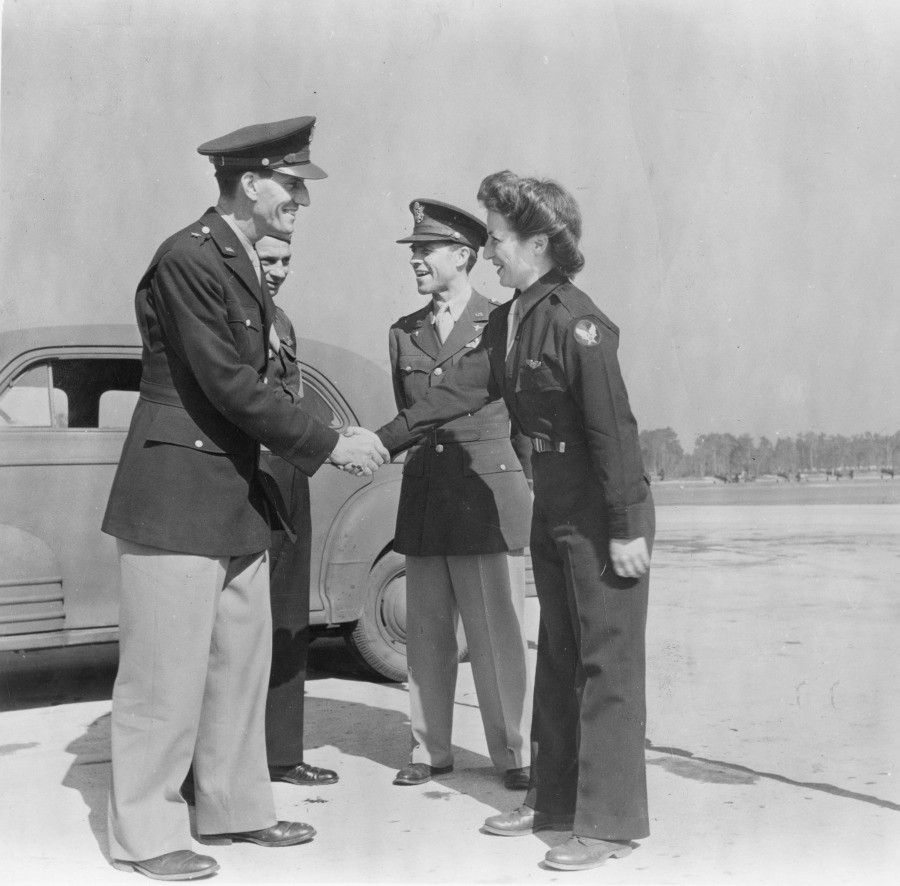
[0,325,469,681]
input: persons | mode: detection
[334,169,656,871]
[180,232,338,809]
[101,116,391,879]
[388,199,535,791]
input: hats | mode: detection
[196,115,329,180]
[395,198,489,252]
[267,231,293,244]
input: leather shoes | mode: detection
[269,763,339,786]
[112,849,221,881]
[543,835,633,871]
[483,804,575,838]
[197,820,317,848]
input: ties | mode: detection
[434,303,454,346]
[504,298,520,363]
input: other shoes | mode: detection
[392,763,454,786]
[504,766,530,790]
